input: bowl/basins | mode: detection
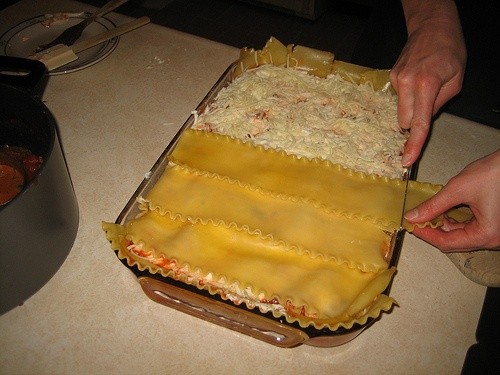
[109,59,411,348]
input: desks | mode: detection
[0,0,500,375]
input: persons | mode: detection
[389,0,500,253]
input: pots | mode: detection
[0,56,80,314]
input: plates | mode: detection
[0,9,120,74]
